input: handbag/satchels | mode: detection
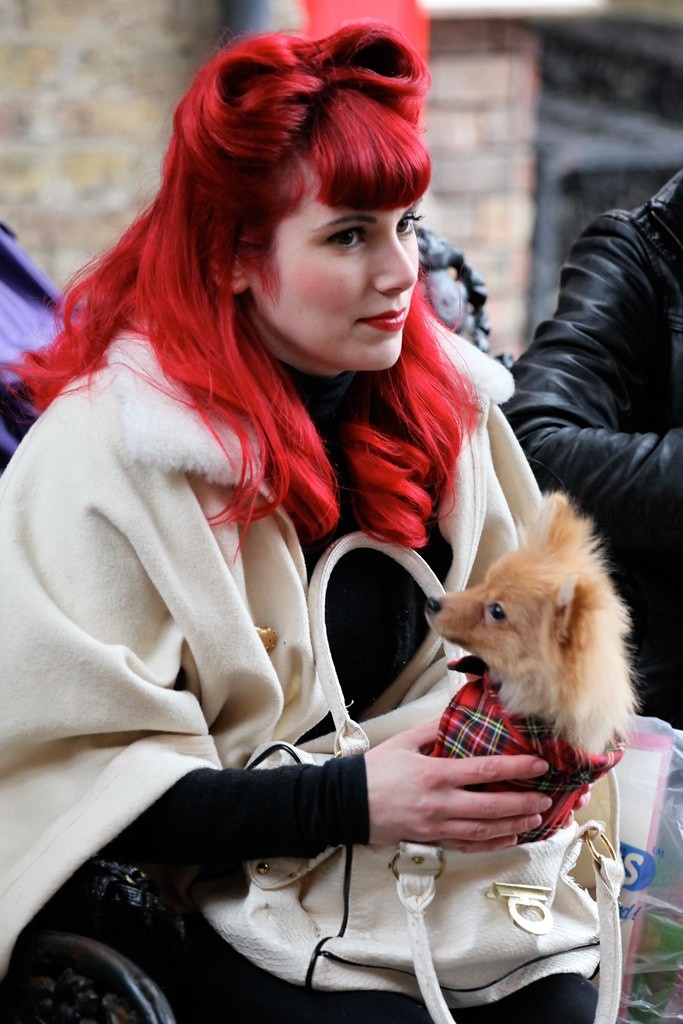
[203,528,623,1024]
[48,856,186,989]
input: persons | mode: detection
[500,168,683,728]
[0,18,598,1024]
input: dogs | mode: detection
[423,485,642,845]
